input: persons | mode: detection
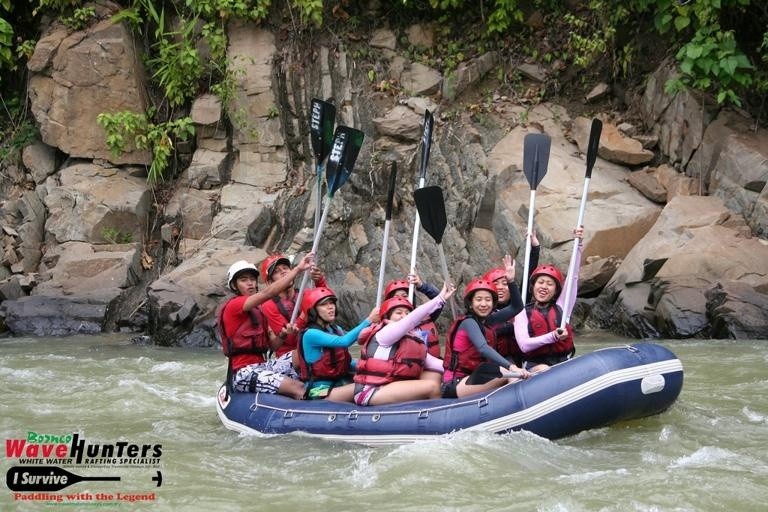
[384,267,444,357]
[482,227,541,356]
[217,252,314,401]
[261,255,325,369]
[513,224,585,372]
[299,286,379,401]
[441,255,532,397]
[354,281,457,406]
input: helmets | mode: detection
[529,265,563,292]
[379,279,416,320]
[303,287,337,313]
[463,267,511,308]
[226,255,290,291]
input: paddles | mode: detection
[521,133,551,306]
[466,362,533,384]
[413,186,458,323]
[290,125,364,327]
[377,161,397,308]
[408,109,434,305]
[309,98,336,289]
[559,119,602,332]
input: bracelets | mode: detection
[278,330,288,341]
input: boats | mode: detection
[215,344,682,442]
